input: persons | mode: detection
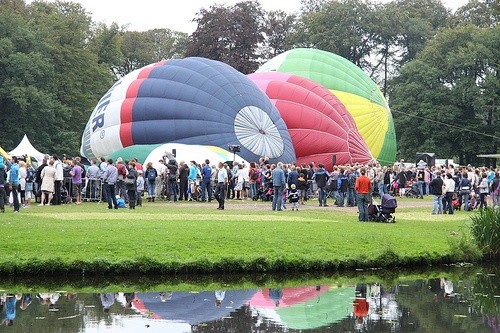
[214,162,228,210]
[355,168,372,222]
[443,173,456,214]
[69,160,84,205]
[0,293,78,312]
[82,155,212,203]
[429,171,443,215]
[0,152,86,214]
[215,290,226,309]
[426,277,453,302]
[126,162,138,209]
[314,163,331,207]
[159,291,172,303]
[329,159,499,207]
[102,159,118,210]
[2,294,16,326]
[123,292,136,310]
[476,172,489,209]
[457,173,472,211]
[269,287,284,307]
[289,184,300,212]
[100,292,115,313]
[353,283,370,329]
[271,162,286,211]
[37,161,57,207]
[20,293,33,311]
[136,170,145,207]
[211,157,331,206]
[489,172,500,207]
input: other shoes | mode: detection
[23,205,31,208]
[0,209,5,213]
[277,209,283,212]
[45,204,51,206]
[272,209,276,211]
[319,204,322,206]
[324,204,329,206]
[292,209,294,211]
[283,206,287,210]
[13,210,20,214]
[37,204,44,207]
[296,209,299,211]
[216,207,225,210]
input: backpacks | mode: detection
[250,169,259,181]
[148,169,156,181]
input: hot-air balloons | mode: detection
[80,57,296,170]
[131,289,260,323]
[245,72,374,175]
[253,47,396,167]
[248,285,330,308]
[254,285,370,329]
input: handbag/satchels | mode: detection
[26,182,33,190]
[124,176,137,185]
[480,187,489,193]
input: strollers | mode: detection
[356,192,397,223]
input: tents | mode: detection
[0,146,10,159]
[7,134,62,199]
